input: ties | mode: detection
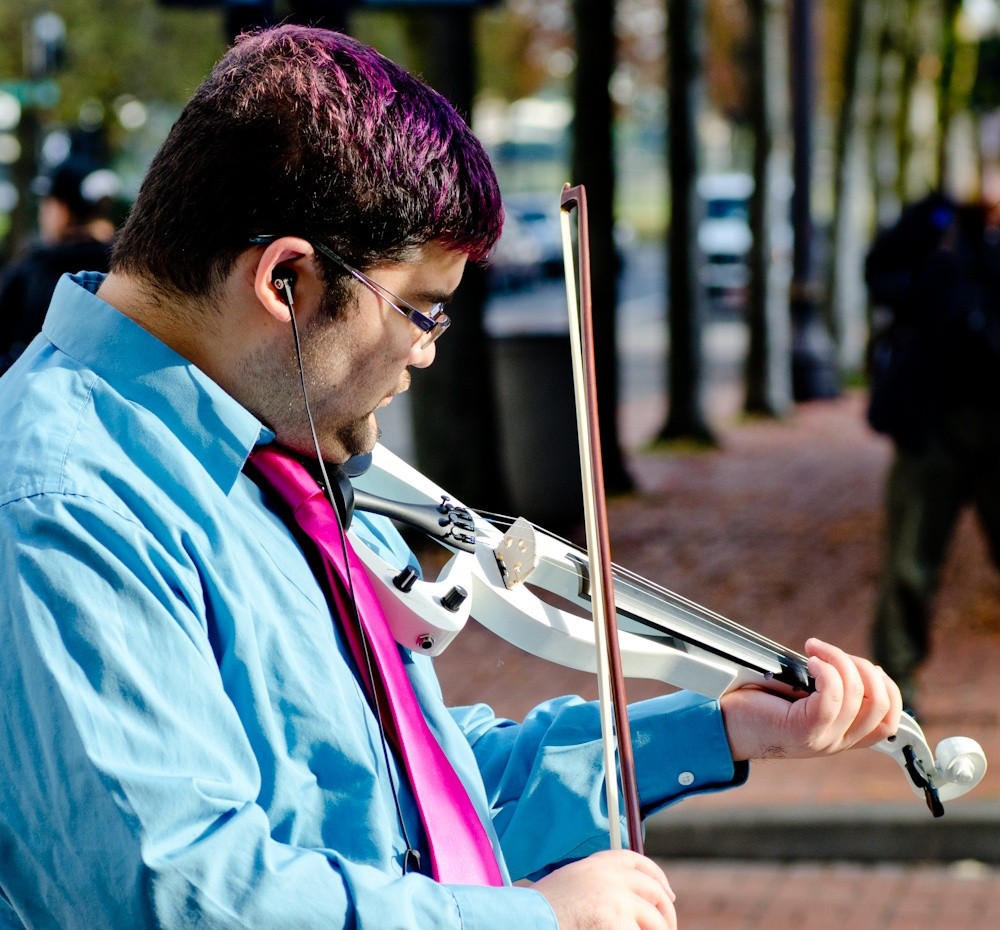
[247,447,506,888]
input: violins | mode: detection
[331,440,992,819]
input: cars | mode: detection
[702,173,758,291]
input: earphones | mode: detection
[272,260,299,308]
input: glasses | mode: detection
[250,233,450,351]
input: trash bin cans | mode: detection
[497,333,592,535]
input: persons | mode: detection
[1,159,999,738]
[1,18,902,930]
